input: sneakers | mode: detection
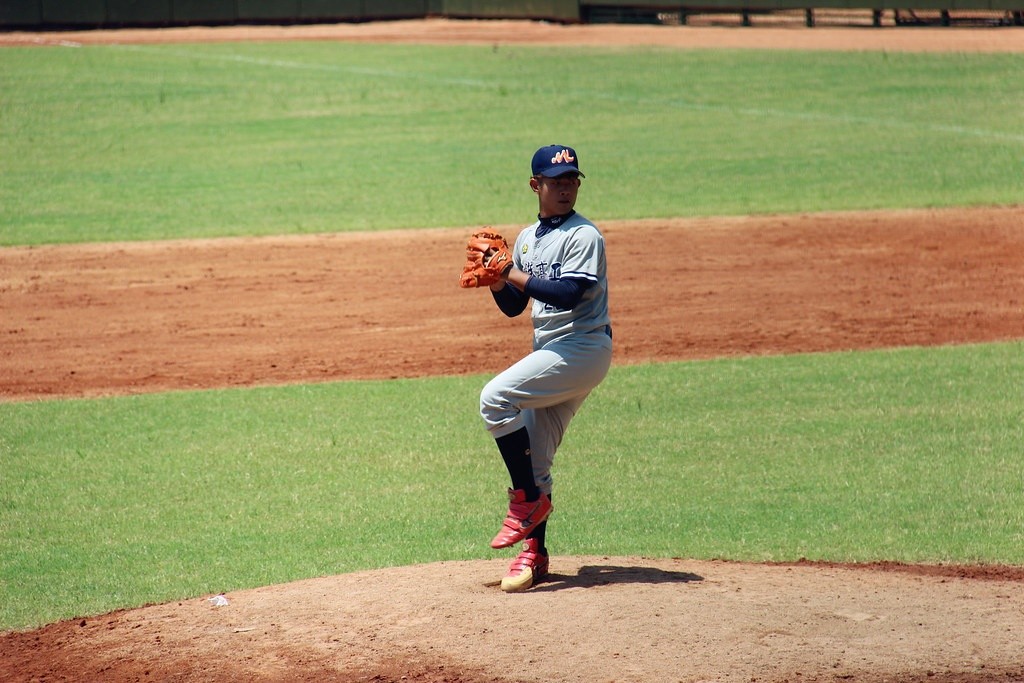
[500,539,549,592]
[490,487,555,548]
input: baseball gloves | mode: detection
[458,224,515,289]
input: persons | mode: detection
[459,142,614,592]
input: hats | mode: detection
[531,144,585,177]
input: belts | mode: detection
[606,326,613,337]
